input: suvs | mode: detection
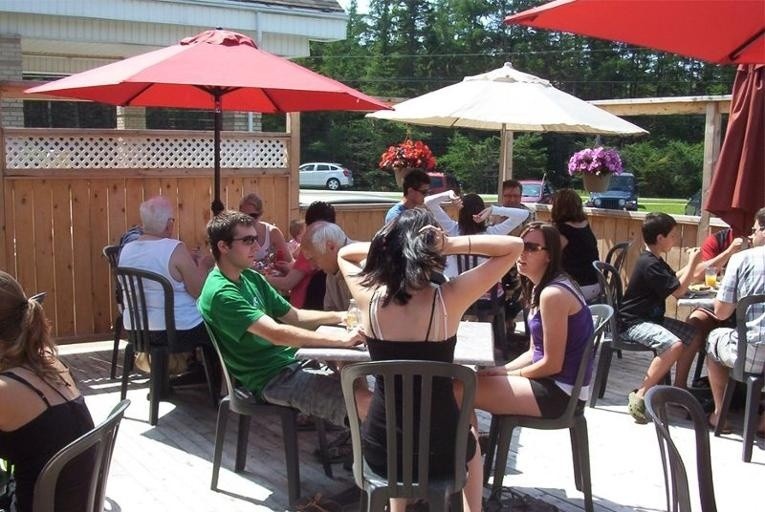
[585,172,638,211]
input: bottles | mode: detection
[346,298,363,335]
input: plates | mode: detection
[688,284,713,295]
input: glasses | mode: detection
[413,187,432,197]
[250,212,260,218]
[224,235,259,246]
[524,241,548,253]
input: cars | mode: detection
[299,161,355,190]
[685,188,702,218]
[519,179,557,203]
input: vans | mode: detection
[425,170,460,199]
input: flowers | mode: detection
[380,139,435,170]
[567,147,621,177]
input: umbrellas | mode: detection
[22,26,396,218]
[364,59,653,205]
[704,63,765,238]
[503,1,764,67]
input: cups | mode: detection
[704,267,717,286]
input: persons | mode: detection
[672,225,746,391]
[239,191,337,310]
[331,207,525,512]
[194,207,375,429]
[113,190,238,393]
[383,169,601,337]
[699,203,765,440]
[1,268,100,511]
[617,210,702,425]
[301,222,363,311]
[447,219,595,512]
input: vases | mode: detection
[393,167,422,189]
[582,168,610,192]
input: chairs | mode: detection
[32,398,129,511]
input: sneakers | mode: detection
[296,411,346,431]
[313,433,364,464]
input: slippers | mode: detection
[707,413,732,434]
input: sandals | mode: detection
[627,389,649,424]
